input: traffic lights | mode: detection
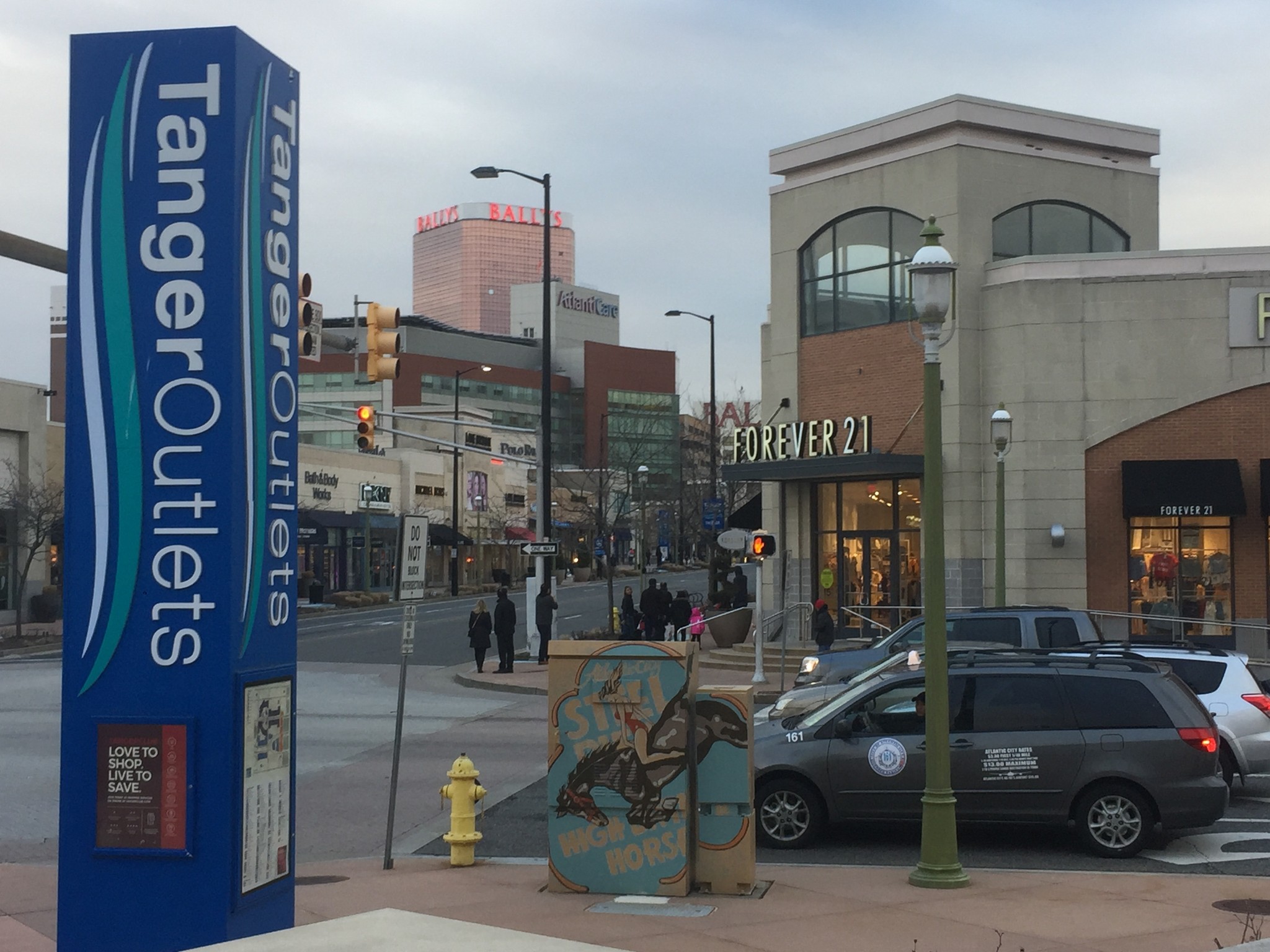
[357,405,374,449]
[298,272,312,356]
[366,303,400,381]
[752,534,776,556]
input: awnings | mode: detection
[428,524,473,546]
[298,509,398,544]
[506,527,535,546]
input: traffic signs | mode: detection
[520,541,559,556]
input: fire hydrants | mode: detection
[611,606,620,633]
[438,752,488,866]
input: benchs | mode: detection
[817,295,918,330]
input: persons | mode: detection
[621,578,705,650]
[535,583,558,665]
[707,565,747,608]
[492,588,516,673]
[468,471,486,511]
[611,548,651,567]
[469,599,492,673]
[911,691,925,736]
[814,599,834,653]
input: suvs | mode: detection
[752,605,1270,858]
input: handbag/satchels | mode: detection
[467,626,473,637]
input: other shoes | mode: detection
[538,657,550,664]
[497,665,514,673]
[492,668,506,675]
[477,666,484,673]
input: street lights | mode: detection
[597,409,625,578]
[908,215,971,888]
[452,365,492,596]
[475,496,482,586]
[989,402,1014,607]
[470,167,552,656]
[637,466,649,593]
[665,311,718,605]
[363,481,372,596]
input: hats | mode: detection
[911,691,926,702]
[816,599,825,610]
[648,578,656,585]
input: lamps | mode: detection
[1051,525,1065,548]
